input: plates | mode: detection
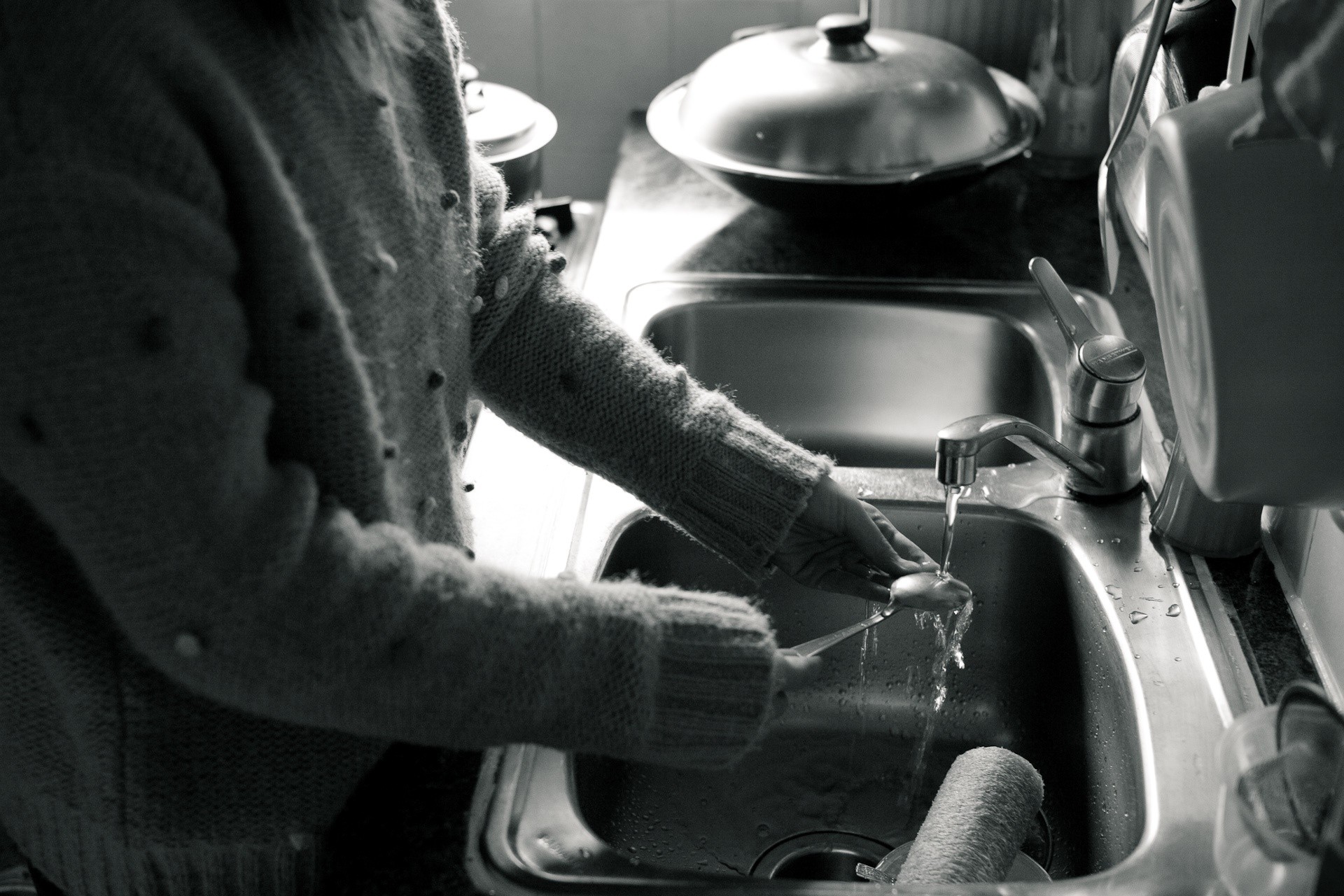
[1022,806,1054,874]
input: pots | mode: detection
[459,61,558,207]
[1107,0,1344,511]
[647,11,1049,220]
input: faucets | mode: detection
[930,256,1155,504]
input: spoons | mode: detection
[788,574,970,658]
[856,861,899,884]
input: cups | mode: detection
[1213,703,1344,896]
[1149,427,1265,557]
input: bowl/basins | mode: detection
[871,834,1055,885]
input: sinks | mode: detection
[609,271,1169,502]
[462,483,1281,896]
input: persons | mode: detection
[0,0,973,894]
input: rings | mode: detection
[863,566,872,579]
